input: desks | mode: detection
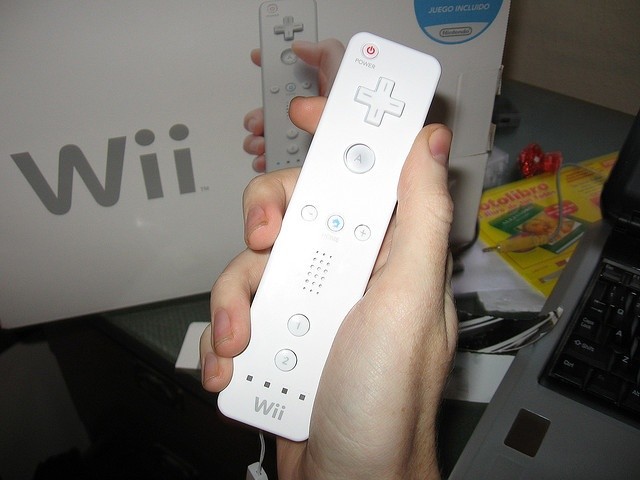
[101,74,639,479]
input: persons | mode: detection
[241,37,346,173]
[197,93,461,479]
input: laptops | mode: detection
[449,110,640,479]
